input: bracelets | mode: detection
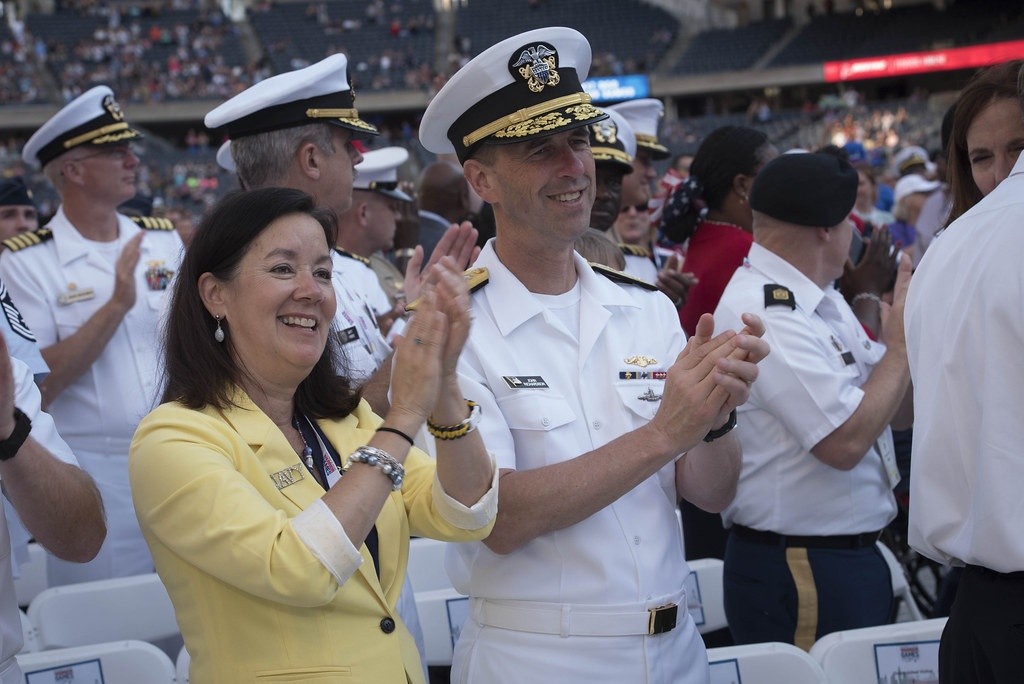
[705,407,739,444]
[345,446,406,492]
[426,398,483,440]
[376,427,415,447]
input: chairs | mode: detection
[13,509,950,684]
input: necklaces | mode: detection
[289,413,316,471]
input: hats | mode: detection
[215,140,235,172]
[587,107,637,174]
[418,26,610,168]
[0,175,37,205]
[894,173,942,201]
[204,53,382,139]
[892,145,930,171]
[843,142,865,161]
[748,153,860,227]
[21,85,145,171]
[353,146,416,203]
[607,98,673,159]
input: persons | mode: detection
[0,0,1024,592]
[126,188,498,684]
[904,148,1024,682]
[382,25,769,684]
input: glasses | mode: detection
[619,200,650,212]
[73,143,134,162]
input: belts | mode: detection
[467,596,688,636]
[731,522,885,550]
